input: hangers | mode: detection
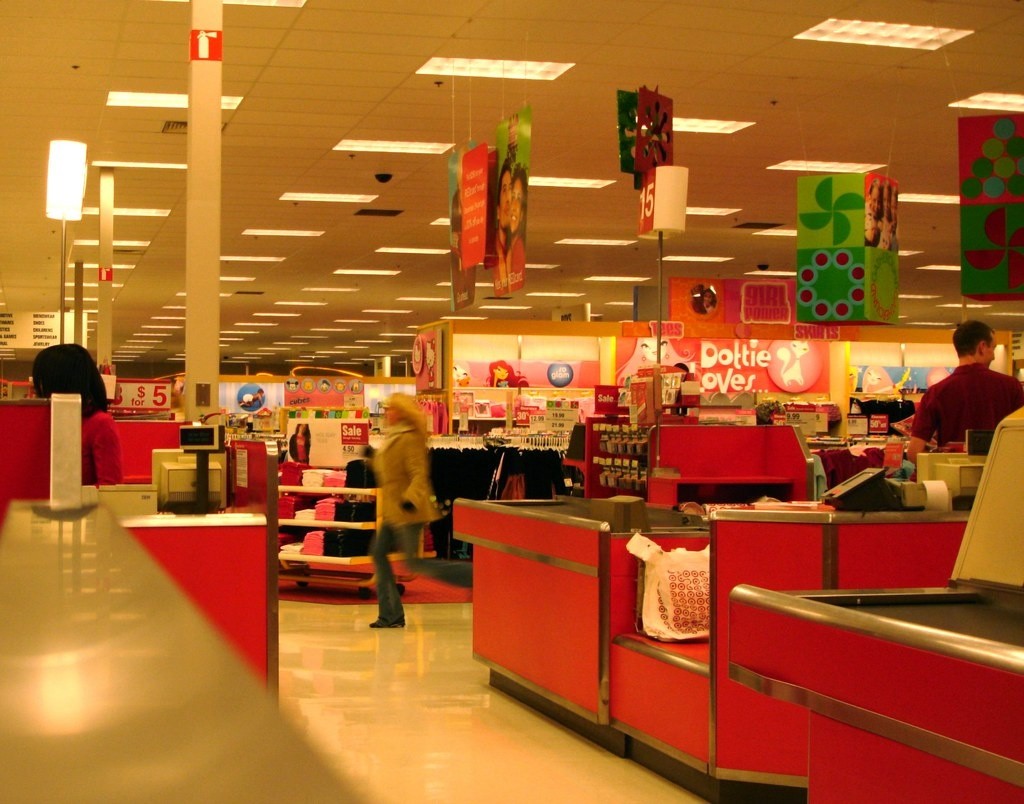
[427,431,572,452]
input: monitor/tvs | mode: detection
[151,450,227,513]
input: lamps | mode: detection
[44,139,87,345]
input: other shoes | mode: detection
[369,614,405,627]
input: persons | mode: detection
[238,388,264,407]
[288,424,312,465]
[906,320,1024,464]
[491,157,528,297]
[865,179,899,253]
[361,392,473,629]
[32,343,123,489]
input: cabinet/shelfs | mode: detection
[277,485,437,567]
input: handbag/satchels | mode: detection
[627,532,710,643]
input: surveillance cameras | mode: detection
[757,265,768,270]
[375,173,392,183]
